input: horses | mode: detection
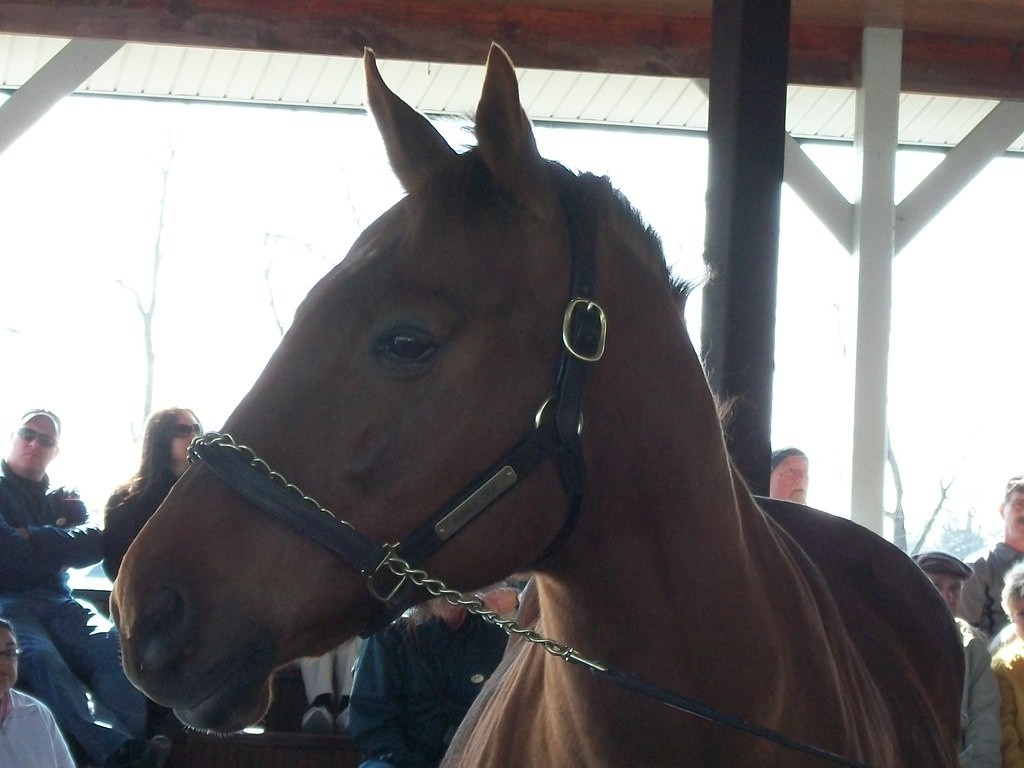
[107,43,964,768]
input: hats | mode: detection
[911,551,974,582]
[21,408,61,437]
[770,448,808,474]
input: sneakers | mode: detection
[104,734,172,768]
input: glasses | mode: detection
[0,649,22,661]
[15,428,59,451]
[169,424,203,438]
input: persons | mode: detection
[0,408,1024,768]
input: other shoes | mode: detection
[336,706,352,737]
[301,706,334,735]
